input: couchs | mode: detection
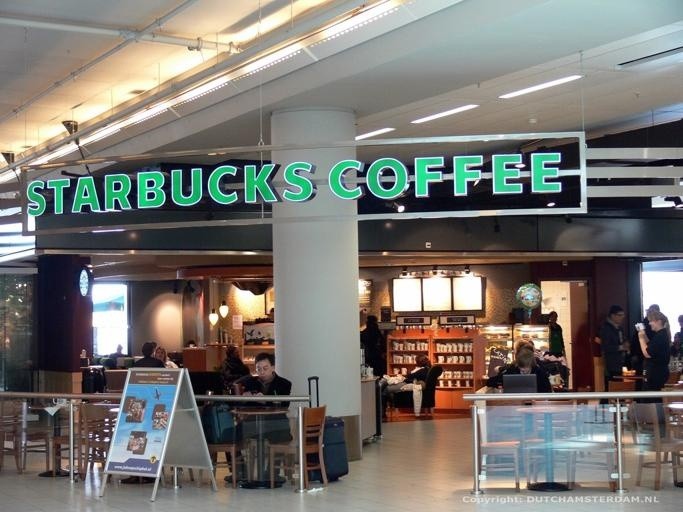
[391,365,443,419]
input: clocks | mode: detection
[78,267,90,298]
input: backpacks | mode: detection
[202,403,237,444]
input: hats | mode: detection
[141,342,157,355]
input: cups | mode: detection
[366,368,373,376]
[232,384,245,396]
[52,398,66,405]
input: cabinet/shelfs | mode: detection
[182,346,238,372]
[485,325,551,363]
[242,321,275,376]
[387,327,485,414]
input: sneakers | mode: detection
[224,472,247,482]
[264,471,285,483]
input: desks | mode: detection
[232,408,289,489]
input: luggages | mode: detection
[308,375,348,482]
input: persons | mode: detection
[134,342,165,367]
[548,311,566,358]
[220,346,250,384]
[126,400,146,422]
[380,354,431,420]
[108,344,127,368]
[486,339,553,393]
[224,353,293,484]
[154,346,180,369]
[593,304,683,439]
[360,315,387,378]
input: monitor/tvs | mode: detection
[187,371,227,395]
[501,373,538,393]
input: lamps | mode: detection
[62,121,91,175]
[544,194,556,207]
[209,279,219,325]
[673,196,683,208]
[219,277,228,319]
[391,200,405,213]
[1,153,21,183]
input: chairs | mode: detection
[168,351,183,368]
[207,444,244,489]
[0,390,123,482]
[477,370,683,492]
[267,405,329,488]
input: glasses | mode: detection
[255,367,272,372]
